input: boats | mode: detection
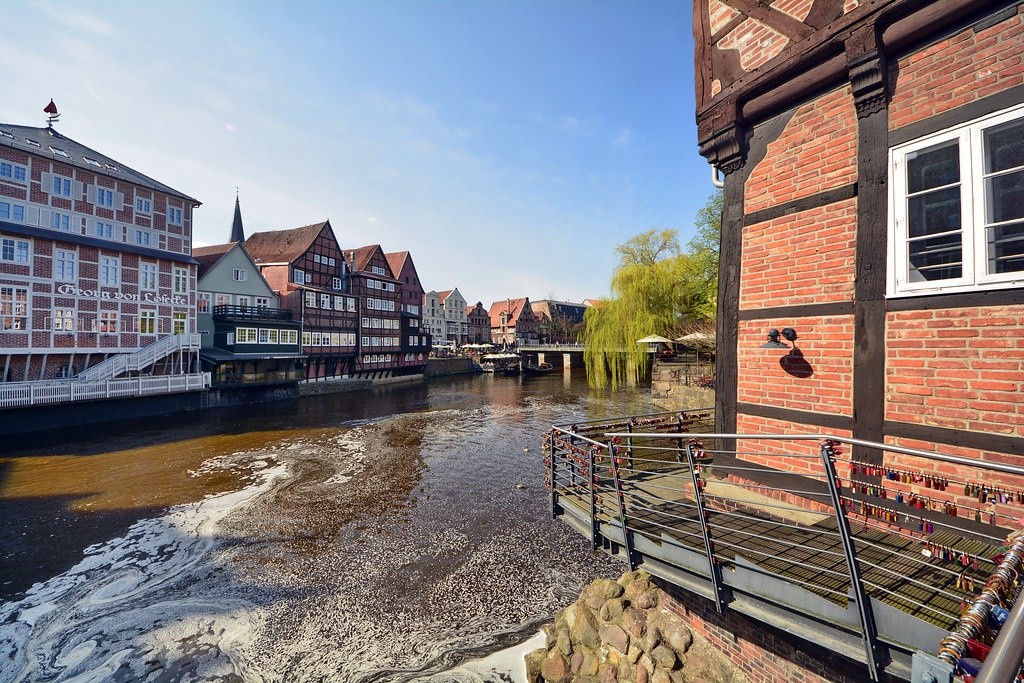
[475,352,521,373]
[521,363,554,374]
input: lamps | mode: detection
[759,328,796,348]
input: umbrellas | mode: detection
[676,332,715,374]
[636,334,678,363]
[431,339,495,354]
